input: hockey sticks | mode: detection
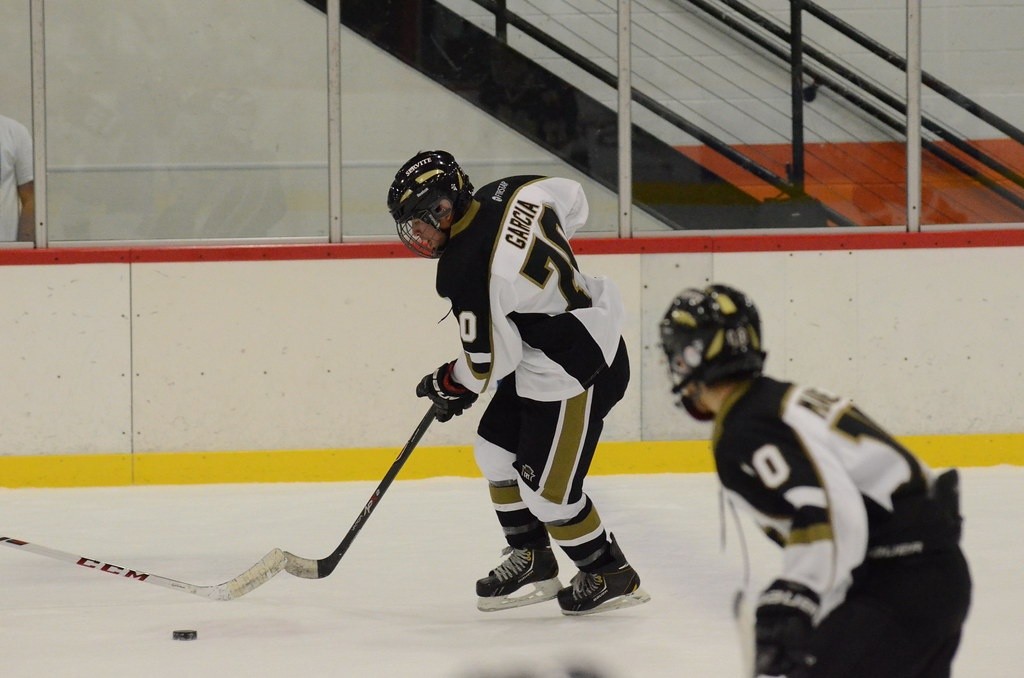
[0,534,287,600]
[282,403,436,579]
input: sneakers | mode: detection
[556,532,651,617]
[476,529,564,612]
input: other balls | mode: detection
[172,630,197,641]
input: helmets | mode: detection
[387,149,474,258]
[658,282,767,420]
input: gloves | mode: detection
[755,613,814,678]
[415,360,479,423]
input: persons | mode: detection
[387,148,635,615]
[658,282,975,677]
[9,117,38,250]
